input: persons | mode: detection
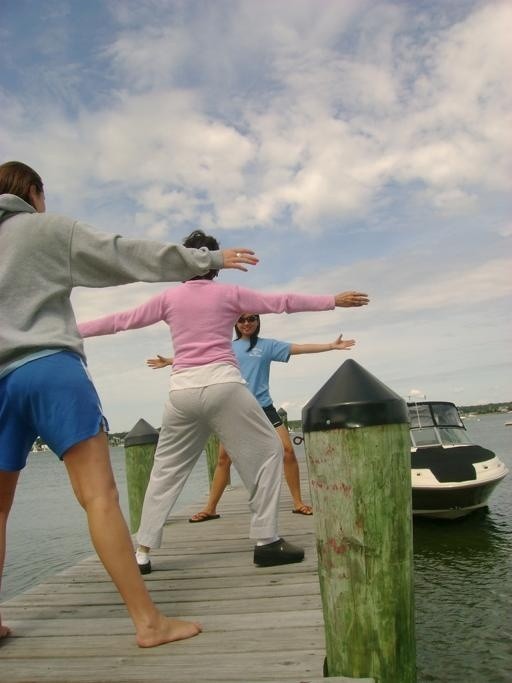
[145,312,356,524]
[76,229,372,575]
[0,160,259,647]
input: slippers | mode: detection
[188,511,220,523]
[291,505,314,515]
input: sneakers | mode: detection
[253,538,305,565]
[133,550,151,575]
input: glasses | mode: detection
[238,317,258,323]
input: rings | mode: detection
[236,252,241,256]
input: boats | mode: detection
[407,402,508,520]
